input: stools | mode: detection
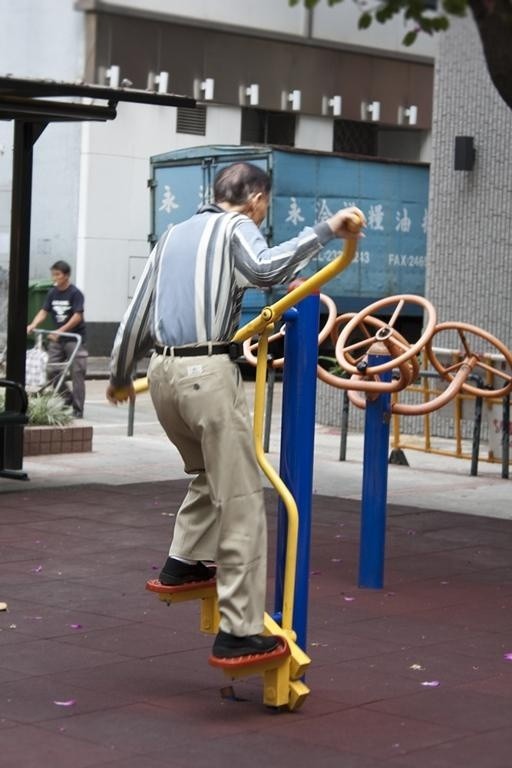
[155,344,231,357]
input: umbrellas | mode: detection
[73,410,82,417]
[63,396,73,409]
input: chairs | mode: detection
[160,557,217,586]
[212,629,279,657]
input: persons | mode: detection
[27,258,89,420]
[104,160,365,658]
